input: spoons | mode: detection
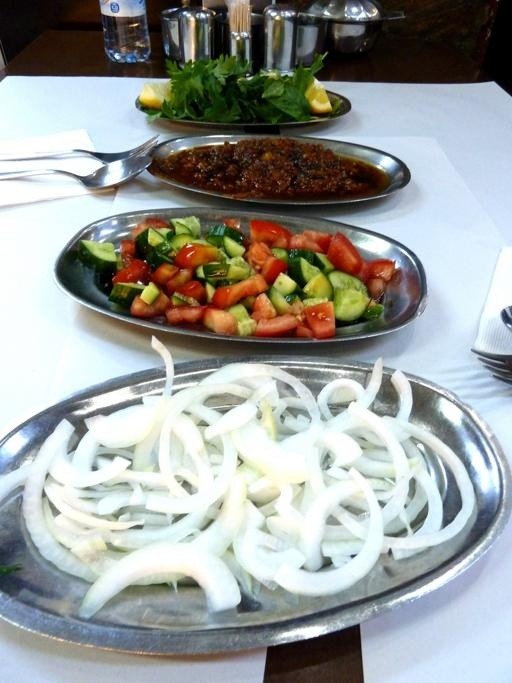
[2,156,156,190]
[499,302,512,333]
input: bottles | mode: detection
[96,0,154,63]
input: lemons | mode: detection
[305,76,332,114]
[139,81,172,108]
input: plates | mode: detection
[50,205,429,346]
[1,358,510,661]
[143,131,412,206]
[135,82,352,129]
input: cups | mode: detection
[162,0,381,70]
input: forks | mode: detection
[4,134,161,157]
[471,344,512,386]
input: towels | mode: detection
[0,129,117,207]
